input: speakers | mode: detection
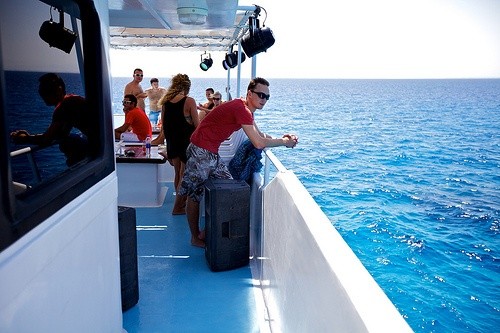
[203,178,250,272]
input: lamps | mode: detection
[241,5,275,59]
[199,51,213,71]
[177,0,208,25]
[222,46,246,71]
[39,6,78,55]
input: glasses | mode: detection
[133,73,144,78]
[252,90,270,100]
[120,100,131,104]
[213,97,221,101]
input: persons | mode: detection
[9,73,89,168]
[178,78,298,248]
[113,69,222,216]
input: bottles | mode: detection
[145,135,151,155]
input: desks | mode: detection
[116,143,169,208]
